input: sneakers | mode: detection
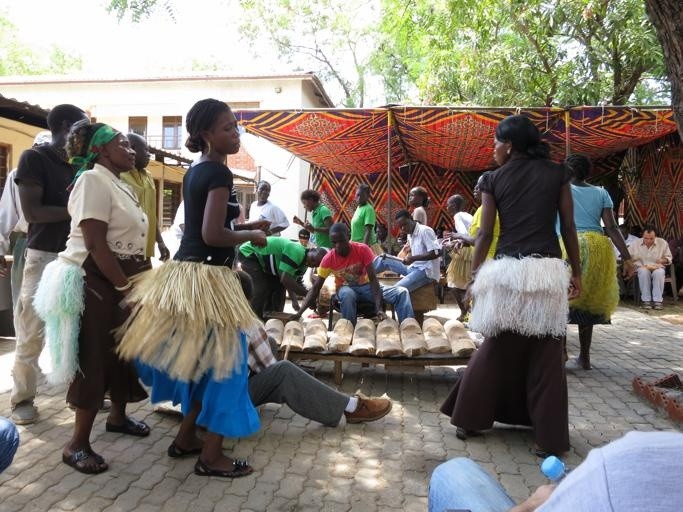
[11,400,35,426]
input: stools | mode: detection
[329,290,380,329]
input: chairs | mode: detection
[630,255,679,308]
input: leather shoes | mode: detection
[344,397,392,424]
[168,439,202,458]
[195,456,253,478]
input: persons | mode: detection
[439,115,580,457]
[603,224,672,310]
[173,201,186,256]
[430,431,683,512]
[233,269,393,427]
[232,180,501,321]
[0,104,170,476]
[556,151,632,370]
[113,99,272,479]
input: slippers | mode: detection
[62,446,109,475]
[105,417,150,438]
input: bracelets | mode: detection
[469,271,478,282]
[115,281,131,291]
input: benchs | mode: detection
[312,272,438,326]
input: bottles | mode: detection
[541,456,576,491]
